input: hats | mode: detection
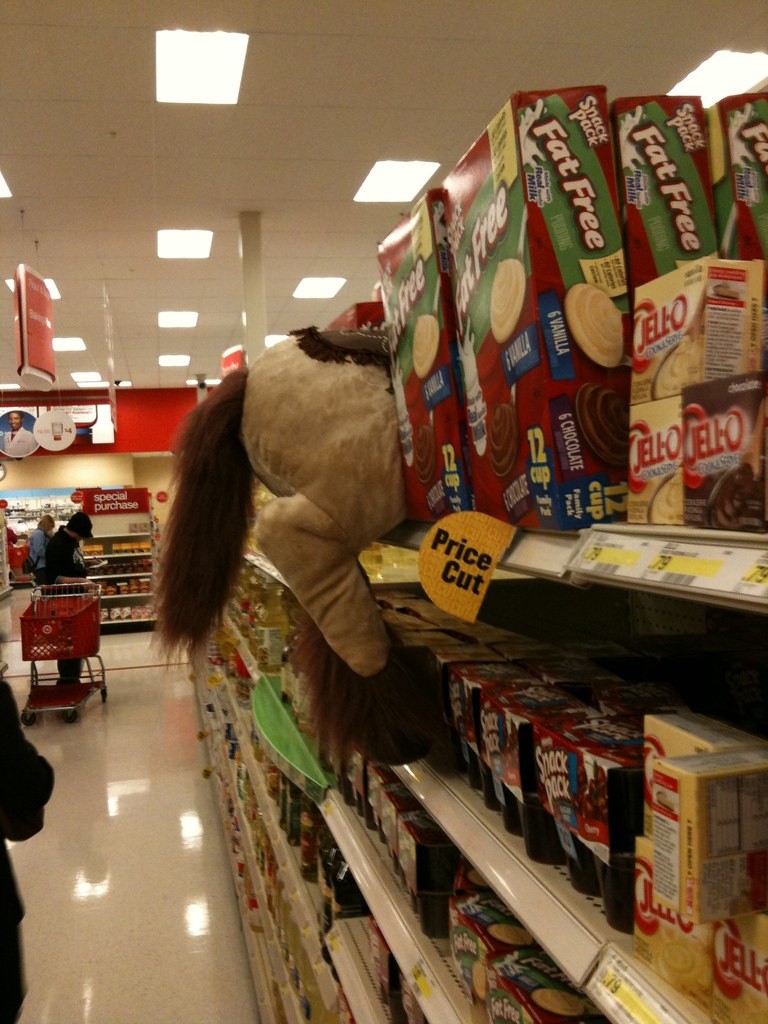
[68,511,93,538]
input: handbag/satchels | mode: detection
[22,555,35,574]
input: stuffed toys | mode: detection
[149,326,448,765]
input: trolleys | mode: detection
[19,583,108,726]
[7,544,36,588]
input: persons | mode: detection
[4,412,38,456]
[0,676,56,1024]
[28,512,99,683]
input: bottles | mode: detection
[195,484,416,1024]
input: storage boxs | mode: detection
[370,93,768,1024]
[82,488,150,537]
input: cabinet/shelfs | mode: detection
[191,527,768,1024]
[80,539,159,626]
[0,528,13,599]
